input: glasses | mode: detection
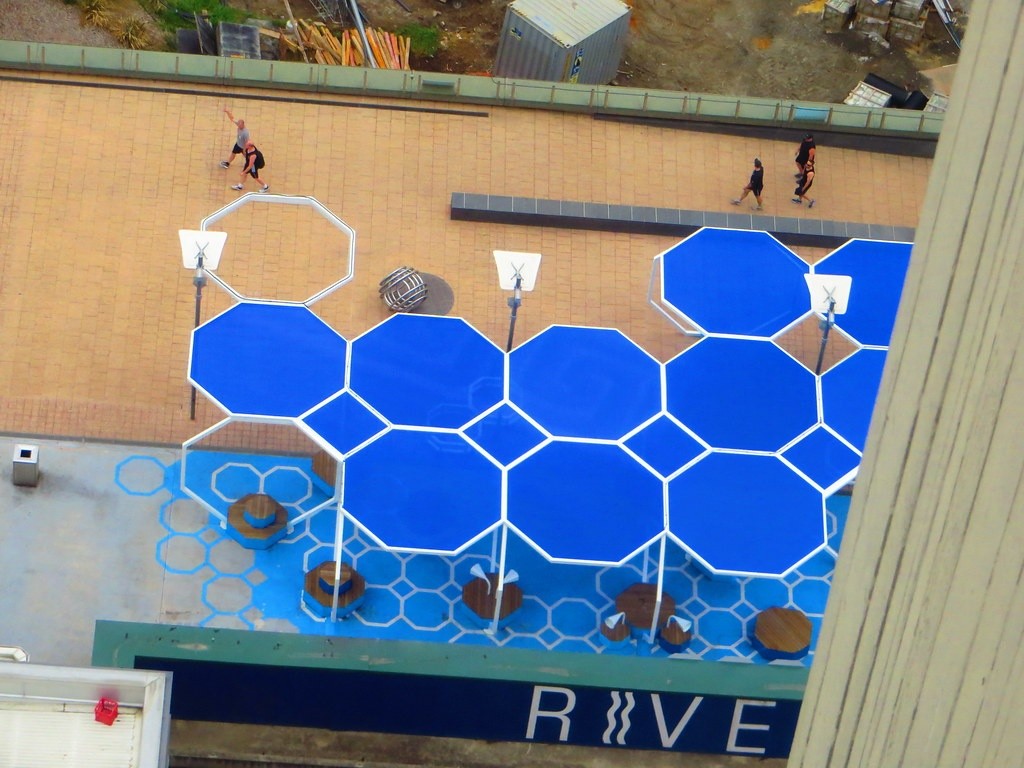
[806,163,812,167]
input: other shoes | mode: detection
[794,173,801,177]
[809,200,816,208]
[796,180,801,184]
[751,205,763,209]
[731,199,742,205]
[792,198,801,204]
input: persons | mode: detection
[218,108,270,193]
[793,132,815,208]
[730,158,764,211]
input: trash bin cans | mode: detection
[11,444,40,486]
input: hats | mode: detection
[802,133,812,139]
[754,158,761,167]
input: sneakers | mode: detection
[232,185,243,190]
[259,185,269,193]
[220,161,229,168]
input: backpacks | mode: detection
[245,146,265,168]
